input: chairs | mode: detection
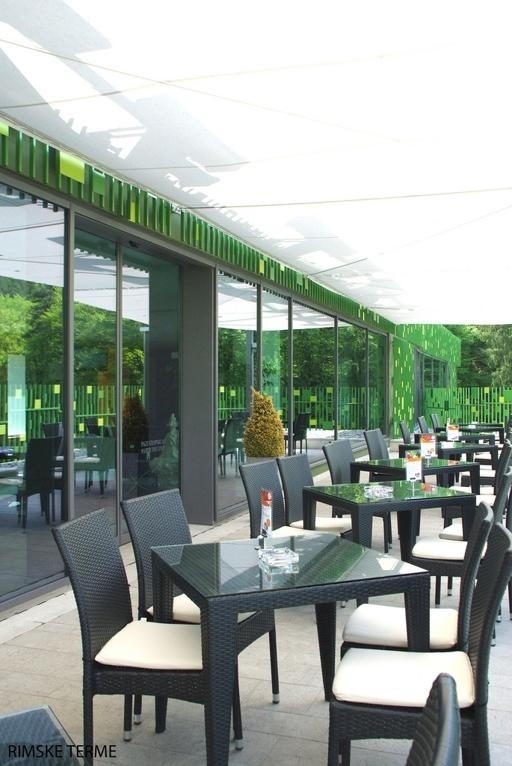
[51,414,512,766]
[0,408,313,529]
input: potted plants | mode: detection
[243,388,286,490]
[119,395,150,498]
